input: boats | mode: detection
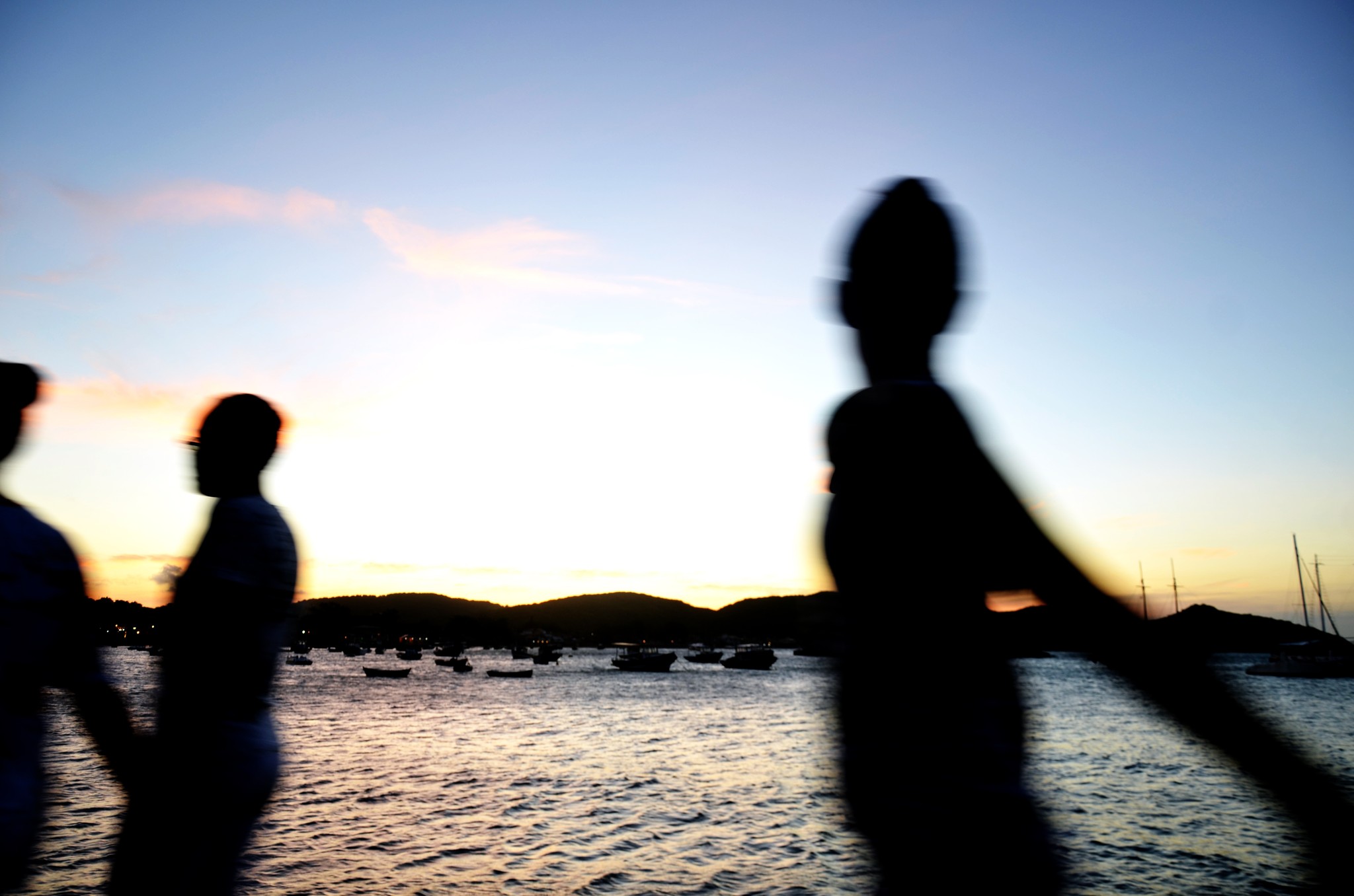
[433,644,474,673]
[289,641,386,657]
[683,642,725,664]
[285,654,313,665]
[127,643,162,657]
[361,664,413,678]
[398,647,424,661]
[610,641,679,672]
[719,643,779,670]
[487,669,535,678]
[507,642,563,666]
[112,643,120,649]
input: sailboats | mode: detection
[1245,530,1354,680]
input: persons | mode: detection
[111,393,298,896]
[823,173,1354,896]
[0,361,171,896]
